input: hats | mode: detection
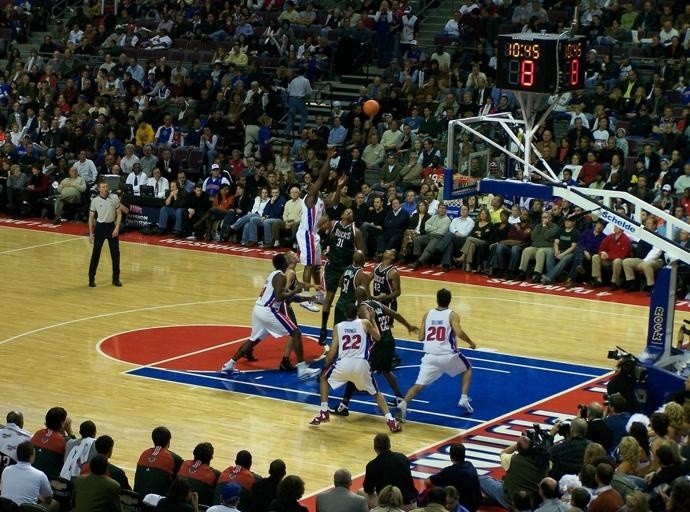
[224,482,241,502]
[211,164,219,170]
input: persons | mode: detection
[0,348,689,512]
[219,244,476,431]
[3,1,689,297]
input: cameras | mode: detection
[602,393,610,406]
[556,419,570,436]
[578,403,587,419]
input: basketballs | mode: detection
[363,101,378,116]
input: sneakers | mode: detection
[300,292,333,312]
[221,365,239,374]
[387,397,406,432]
[89,282,95,287]
[113,280,122,286]
[312,406,349,424]
[458,399,473,413]
[414,259,654,291]
[243,351,256,361]
[280,363,322,381]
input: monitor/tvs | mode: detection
[122,184,134,196]
[140,185,155,197]
[165,189,185,199]
